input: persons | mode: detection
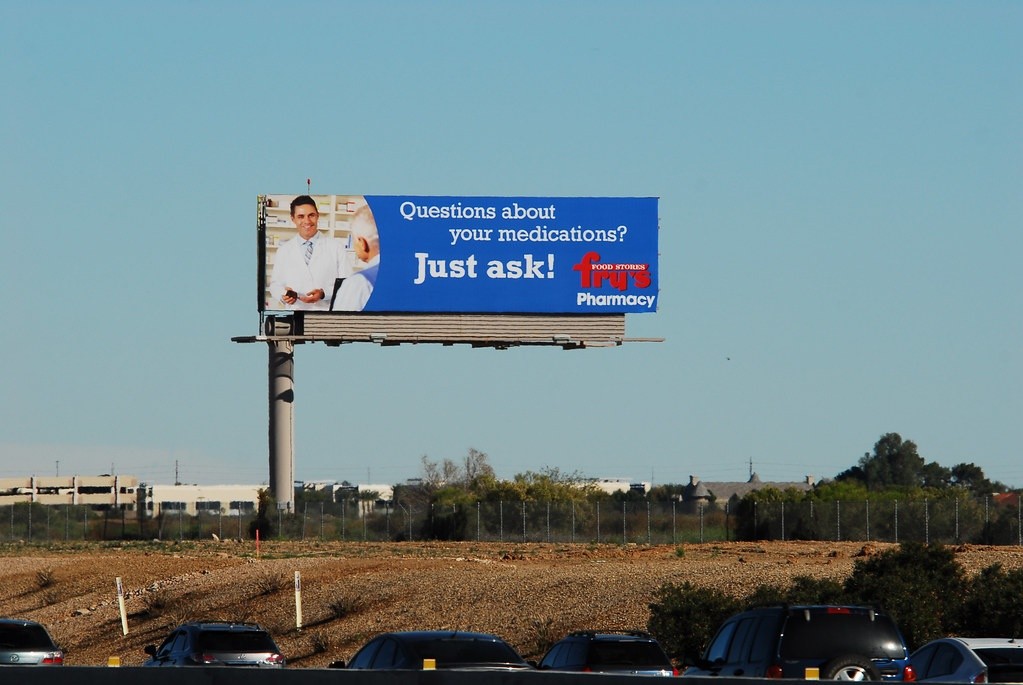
[269,195,349,311]
[332,205,380,311]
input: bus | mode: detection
[681,606,917,684]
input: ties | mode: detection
[304,242,313,266]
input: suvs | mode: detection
[529,630,677,678]
[144,621,286,669]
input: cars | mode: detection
[910,637,1023,683]
[0,619,64,665]
[329,632,537,670]
